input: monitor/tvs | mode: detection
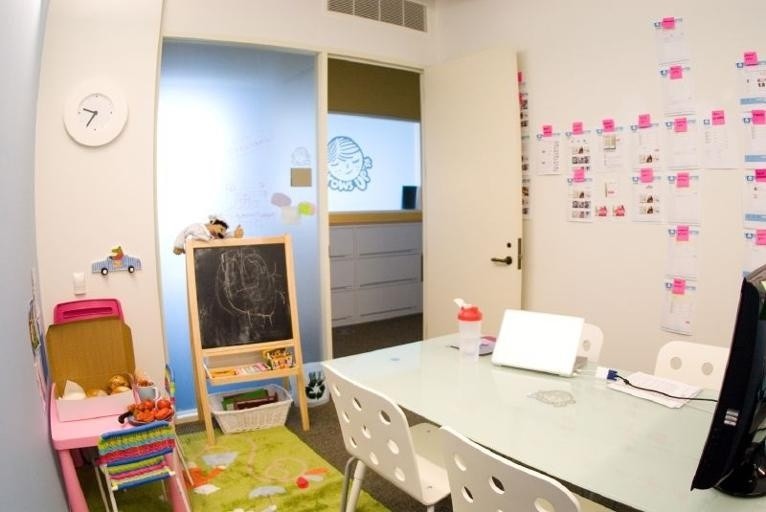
[688,262,765,497]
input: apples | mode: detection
[128,399,171,418]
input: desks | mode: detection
[48,379,191,511]
[325,330,766,512]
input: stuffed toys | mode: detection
[173,216,244,255]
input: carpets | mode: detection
[66,423,390,512]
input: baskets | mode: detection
[207,384,293,434]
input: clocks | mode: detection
[62,78,128,148]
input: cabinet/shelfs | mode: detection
[354,222,422,325]
[329,226,354,329]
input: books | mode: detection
[220,387,280,412]
[210,361,272,379]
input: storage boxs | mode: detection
[44,316,136,422]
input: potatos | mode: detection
[86,375,130,398]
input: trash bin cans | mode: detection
[288,361,330,408]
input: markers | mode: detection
[209,363,267,378]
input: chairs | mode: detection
[91,422,193,512]
[654,341,732,394]
[574,324,604,374]
[438,427,618,512]
[322,364,451,512]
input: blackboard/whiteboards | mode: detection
[185,234,299,357]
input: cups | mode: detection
[137,382,160,402]
[459,302,483,366]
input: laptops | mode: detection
[491,307,590,377]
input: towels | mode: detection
[608,371,702,409]
